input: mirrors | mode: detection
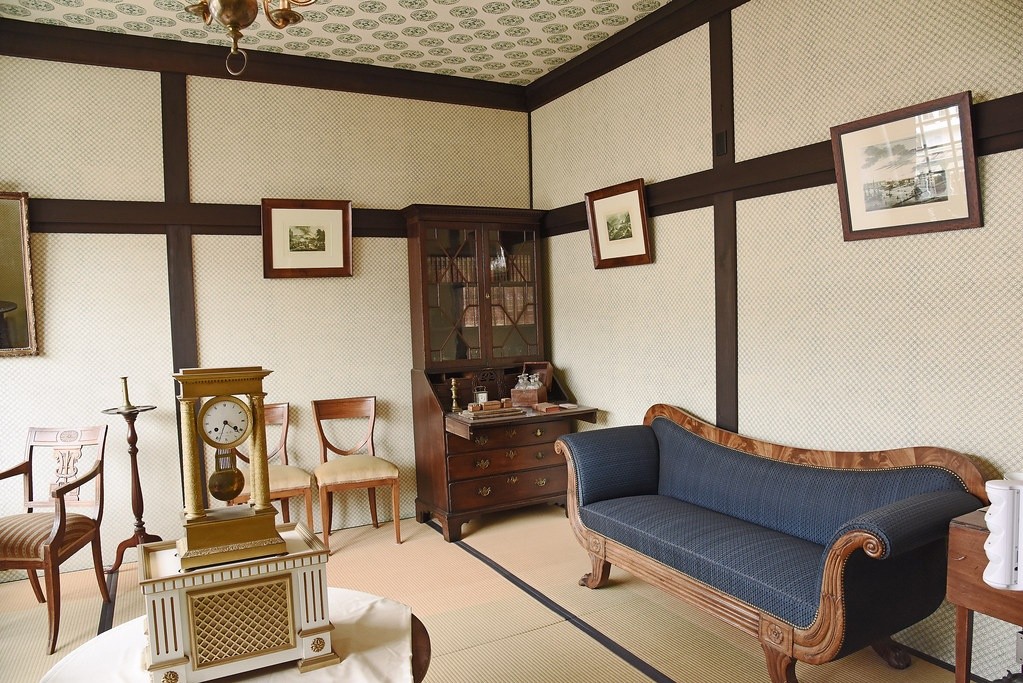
[0,190,38,358]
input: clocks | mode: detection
[473,386,489,406]
[165,365,288,572]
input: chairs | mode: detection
[0,424,112,655]
[227,402,314,533]
[310,395,402,556]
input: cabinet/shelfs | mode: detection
[397,200,599,544]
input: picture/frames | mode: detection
[258,197,353,278]
[583,178,655,271]
[829,89,983,241]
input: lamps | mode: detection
[182,0,316,78]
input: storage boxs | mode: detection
[511,360,553,407]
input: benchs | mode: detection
[554,402,990,683]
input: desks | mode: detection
[38,586,431,683]
[0,301,18,349]
[945,506,1023,683]
